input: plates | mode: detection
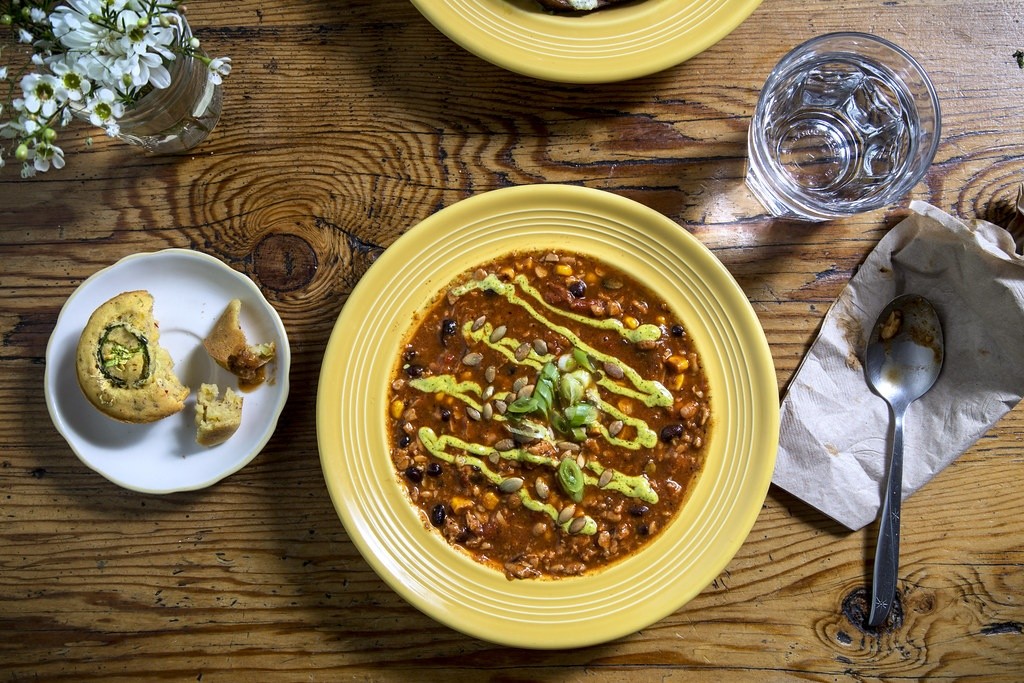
[316,184,780,650]
[411,0,764,83]
[43,248,289,493]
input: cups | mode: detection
[743,32,939,221]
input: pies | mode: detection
[75,289,274,445]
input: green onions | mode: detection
[508,362,589,501]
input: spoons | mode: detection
[863,293,946,635]
